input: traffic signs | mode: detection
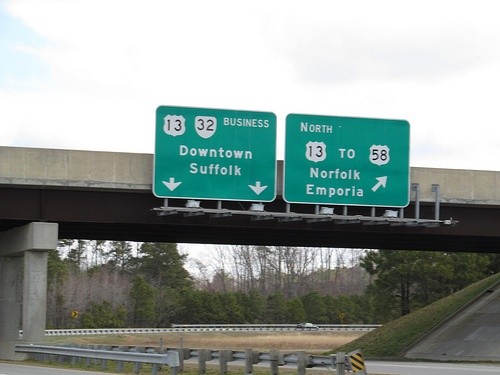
[151,104,278,202]
[282,113,411,209]
